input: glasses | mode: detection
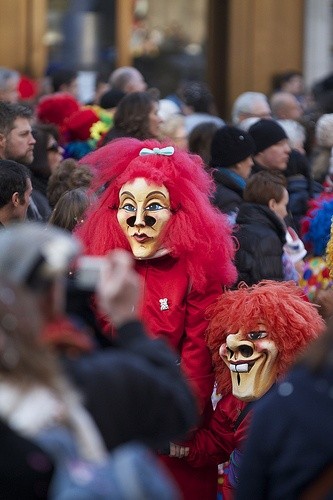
[47,142,60,152]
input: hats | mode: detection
[37,94,79,124]
[156,100,178,123]
[186,112,224,138]
[208,126,252,169]
[316,113,333,148]
[59,107,108,146]
[17,74,38,101]
[45,59,78,88]
[245,118,288,155]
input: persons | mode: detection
[0,279,115,500]
[0,157,34,224]
[49,186,99,234]
[26,121,66,222]
[47,156,103,208]
[231,167,291,288]
[243,117,289,189]
[0,56,333,177]
[73,143,235,500]
[228,319,333,500]
[204,123,253,214]
[284,149,324,227]
[163,280,326,499]
[0,223,199,462]
